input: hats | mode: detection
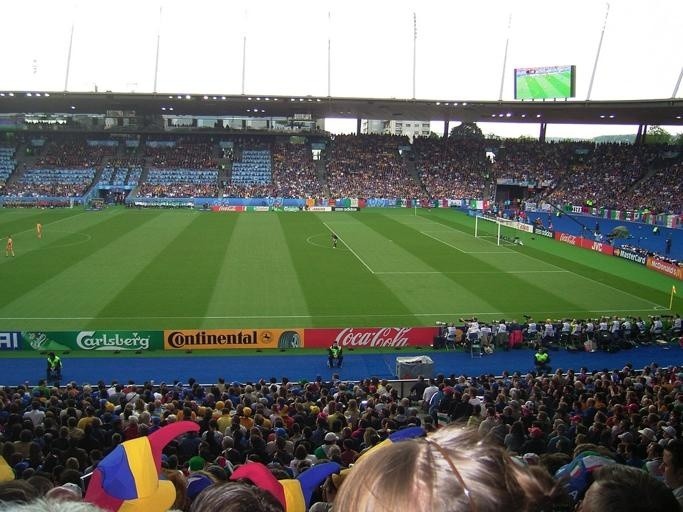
[324,432,340,441]
[618,426,678,441]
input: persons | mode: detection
[4,234,15,257]
[436,311,681,362]
[330,234,337,249]
[617,238,682,269]
[327,340,343,369]
[46,352,62,381]
[0,361,683,511]
[2,119,682,236]
[35,221,42,241]
